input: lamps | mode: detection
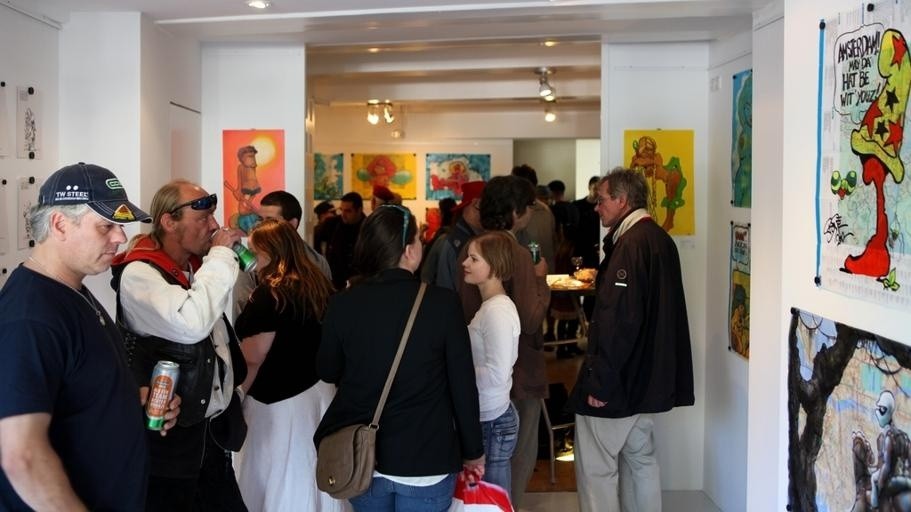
[532,67,561,103]
[545,105,560,123]
[365,99,395,125]
[392,104,409,140]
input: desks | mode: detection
[538,275,596,349]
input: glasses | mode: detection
[527,200,536,208]
[378,204,409,248]
[163,193,217,210]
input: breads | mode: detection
[550,268,598,290]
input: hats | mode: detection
[315,201,338,215]
[38,162,153,225]
[373,185,395,202]
[452,182,487,212]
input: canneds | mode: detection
[144,360,180,431]
[527,239,541,264]
[210,229,259,274]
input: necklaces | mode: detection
[28,254,106,328]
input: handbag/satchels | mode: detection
[316,425,376,499]
[447,468,514,512]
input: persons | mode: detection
[109,177,248,512]
[0,160,182,512]
[232,162,698,511]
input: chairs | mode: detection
[540,362,577,487]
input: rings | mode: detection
[222,226,231,232]
